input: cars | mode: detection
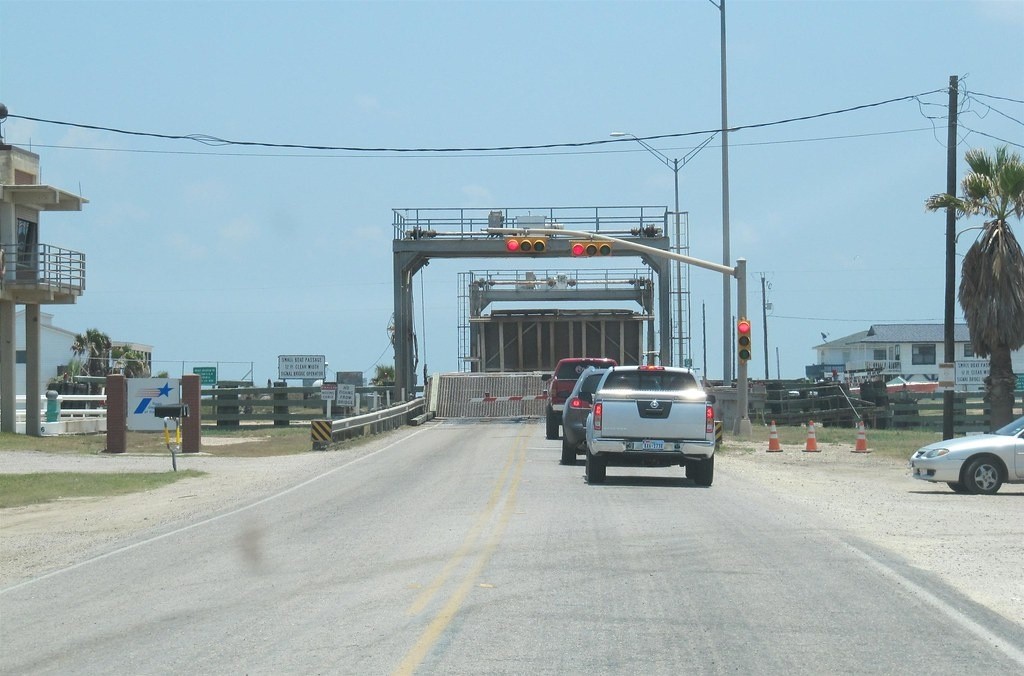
[908,414,1024,495]
[585,364,722,490]
[560,364,607,466]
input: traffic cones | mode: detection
[852,421,872,454]
[765,419,784,453]
[802,419,822,454]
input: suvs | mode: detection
[540,352,621,440]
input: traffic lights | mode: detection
[505,236,547,254]
[569,239,614,257]
[736,318,751,361]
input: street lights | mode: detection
[605,124,754,366]
